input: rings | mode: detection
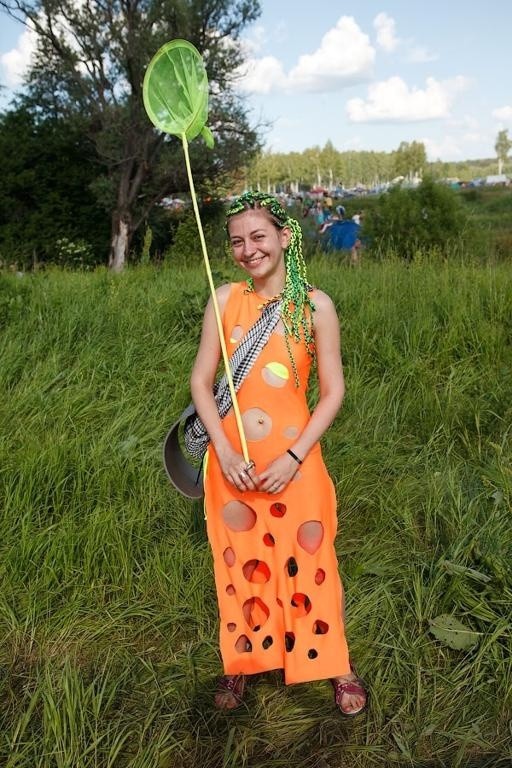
[243,460,254,472]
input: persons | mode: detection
[323,192,332,208]
[335,183,356,199]
[160,196,184,210]
[278,192,292,206]
[189,189,369,716]
[304,202,362,258]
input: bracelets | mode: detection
[285,448,303,464]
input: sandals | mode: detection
[214,672,248,710]
[330,657,368,716]
